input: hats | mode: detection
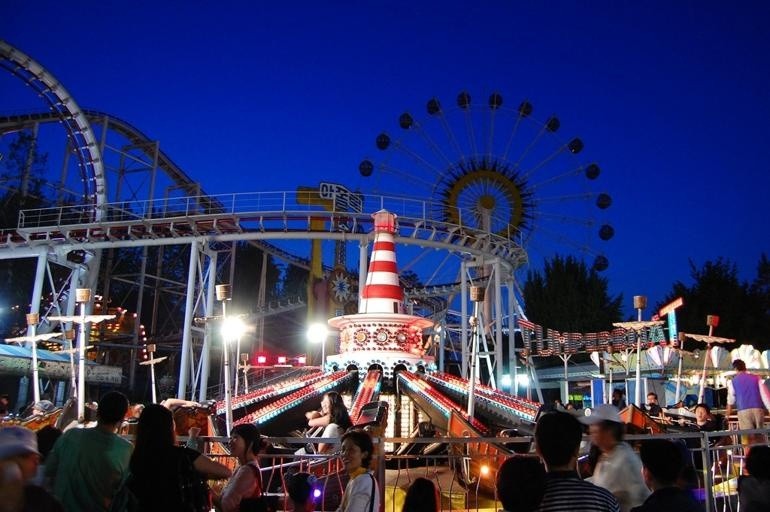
[32,400,53,412]
[576,404,622,425]
[285,471,318,503]
[0,426,43,458]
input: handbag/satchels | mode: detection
[216,496,279,512]
[180,475,209,512]
[112,486,138,512]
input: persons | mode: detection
[1,360,770,511]
